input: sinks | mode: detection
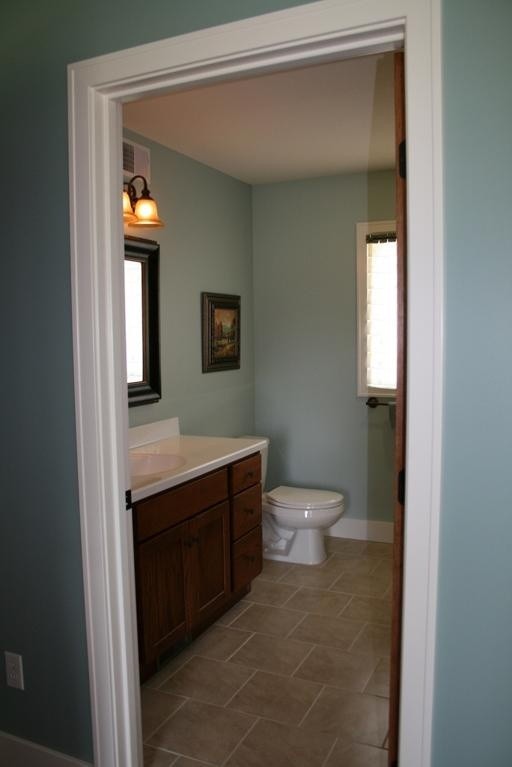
[130,450,187,475]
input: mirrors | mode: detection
[123,235,162,408]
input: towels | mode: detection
[389,402,396,429]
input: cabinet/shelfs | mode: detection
[232,447,263,602]
[133,463,232,686]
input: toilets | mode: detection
[237,433,348,567]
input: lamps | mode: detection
[123,175,165,228]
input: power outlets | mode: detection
[4,652,24,690]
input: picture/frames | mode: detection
[202,290,241,373]
